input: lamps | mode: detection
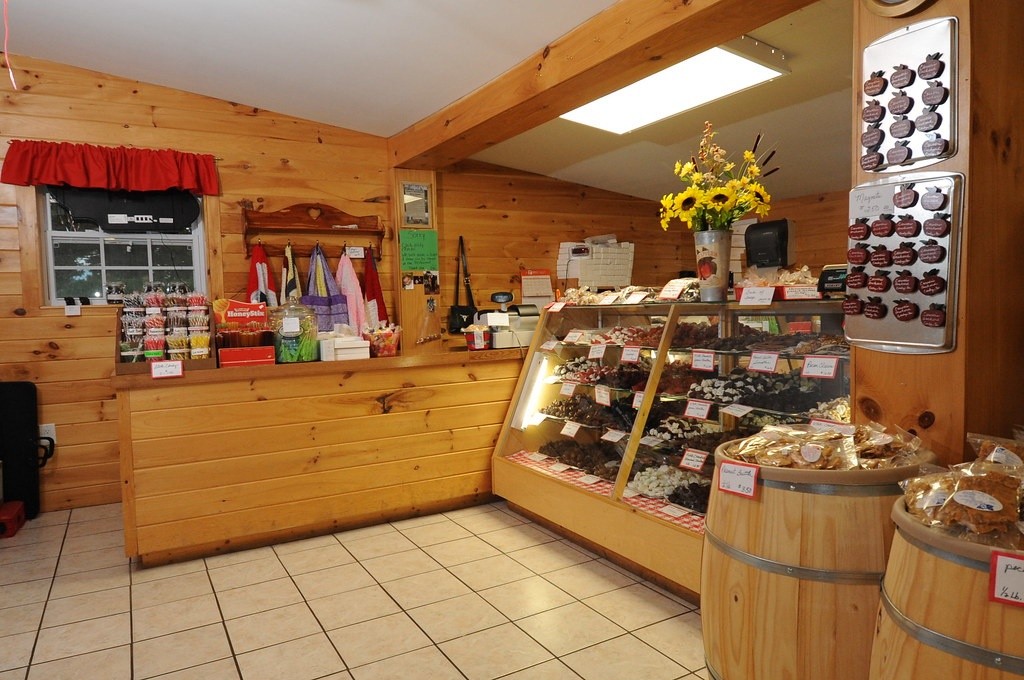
[558,34,792,135]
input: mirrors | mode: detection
[400,181,433,229]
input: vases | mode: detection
[693,234,735,303]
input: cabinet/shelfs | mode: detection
[114,293,218,375]
[493,298,855,608]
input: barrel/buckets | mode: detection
[865,494,1024,680]
[699,436,919,680]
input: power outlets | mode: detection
[39,424,58,446]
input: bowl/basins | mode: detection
[462,330,490,351]
[363,332,399,357]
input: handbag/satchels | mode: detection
[448,304,477,335]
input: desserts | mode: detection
[536,314,849,513]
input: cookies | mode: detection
[726,423,1024,552]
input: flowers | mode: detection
[658,122,780,231]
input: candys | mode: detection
[119,290,212,361]
[364,327,400,357]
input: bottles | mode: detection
[107,280,211,361]
[269,295,319,362]
